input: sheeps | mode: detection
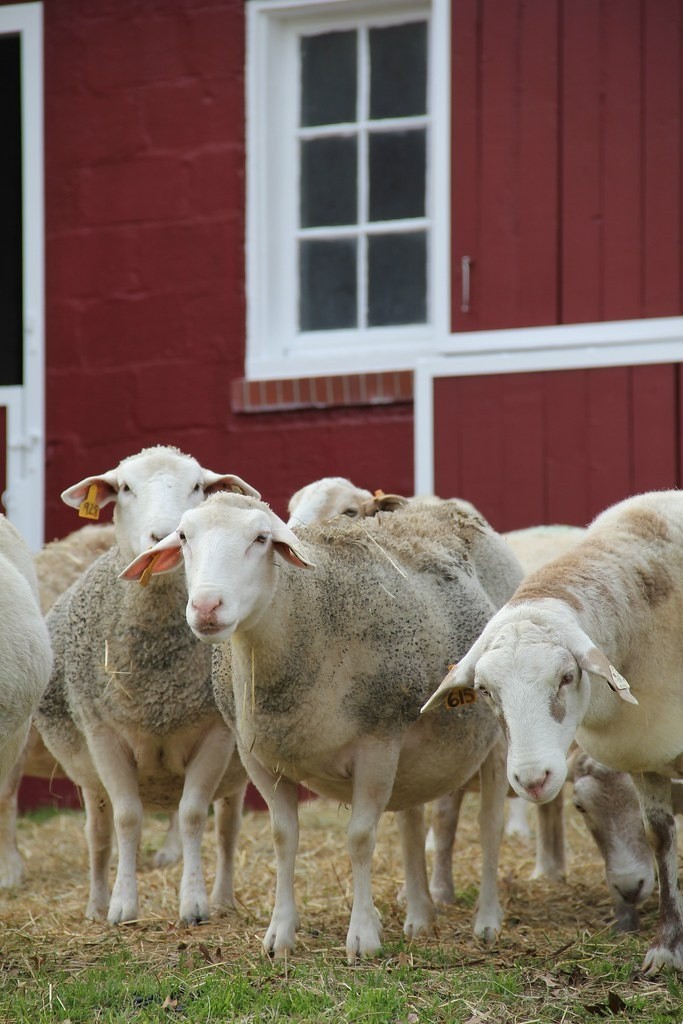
[0,444,683,983]
